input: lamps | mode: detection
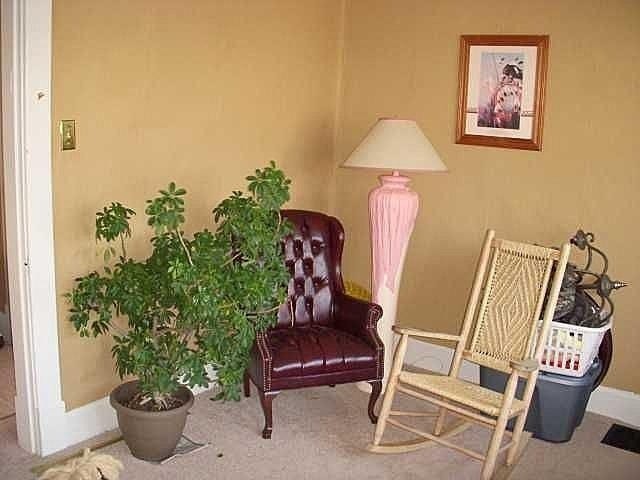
[337,119,449,395]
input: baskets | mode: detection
[530,311,613,378]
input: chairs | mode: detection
[364,229,572,480]
[228,207,386,440]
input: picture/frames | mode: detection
[455,32,552,152]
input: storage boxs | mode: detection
[478,360,601,444]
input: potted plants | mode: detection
[62,160,295,462]
